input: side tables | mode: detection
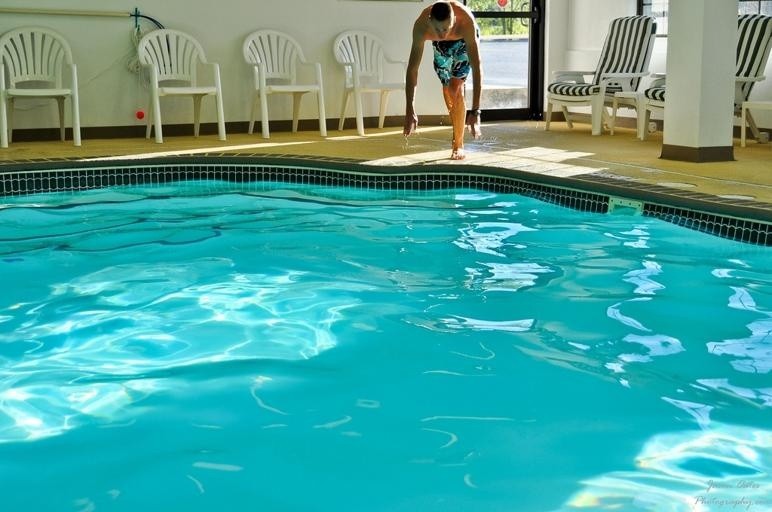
[610,91,642,139]
[741,101,772,147]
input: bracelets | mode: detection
[468,109,482,116]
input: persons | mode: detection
[403,0,484,160]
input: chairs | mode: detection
[138,28,226,143]
[545,15,656,136]
[244,30,327,137]
[0,26,81,148]
[334,30,408,136]
[641,14,771,143]
